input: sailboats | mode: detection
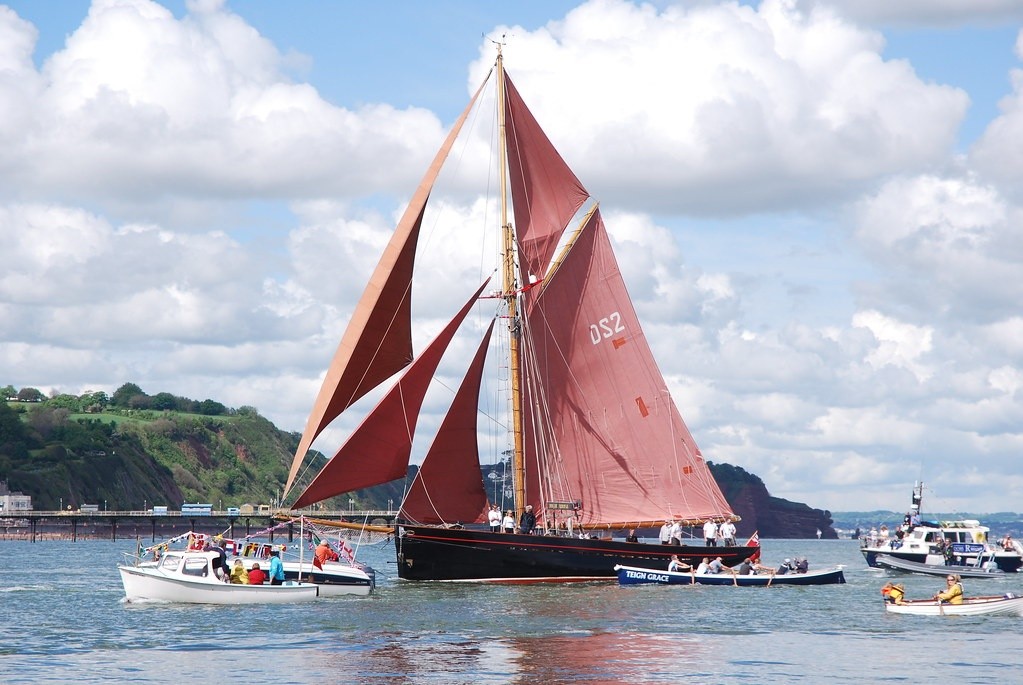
[280,30,763,580]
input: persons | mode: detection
[626,528,638,543]
[231,559,249,584]
[719,517,737,547]
[865,510,923,551]
[203,540,230,579]
[659,518,672,545]
[703,515,719,546]
[937,573,964,604]
[316,540,339,564]
[670,520,683,546]
[934,536,954,565]
[882,582,906,605]
[488,504,502,533]
[669,554,811,576]
[268,549,285,585]
[519,505,536,534]
[249,563,266,585]
[503,509,516,533]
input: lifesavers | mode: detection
[977,532,984,542]
[191,534,204,550]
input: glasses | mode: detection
[947,578,954,581]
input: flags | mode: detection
[188,532,274,560]
[337,536,353,559]
[312,553,324,571]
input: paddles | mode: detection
[937,597,942,617]
[767,573,774,587]
[690,565,695,584]
[731,567,738,586]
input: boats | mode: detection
[614,564,845,586]
[858,483,1023,572]
[135,532,376,597]
[117,549,319,605]
[876,543,1005,579]
[886,595,1023,615]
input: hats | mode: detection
[526,505,532,510]
[252,563,260,569]
[321,540,328,546]
[881,525,888,529]
[745,558,750,563]
